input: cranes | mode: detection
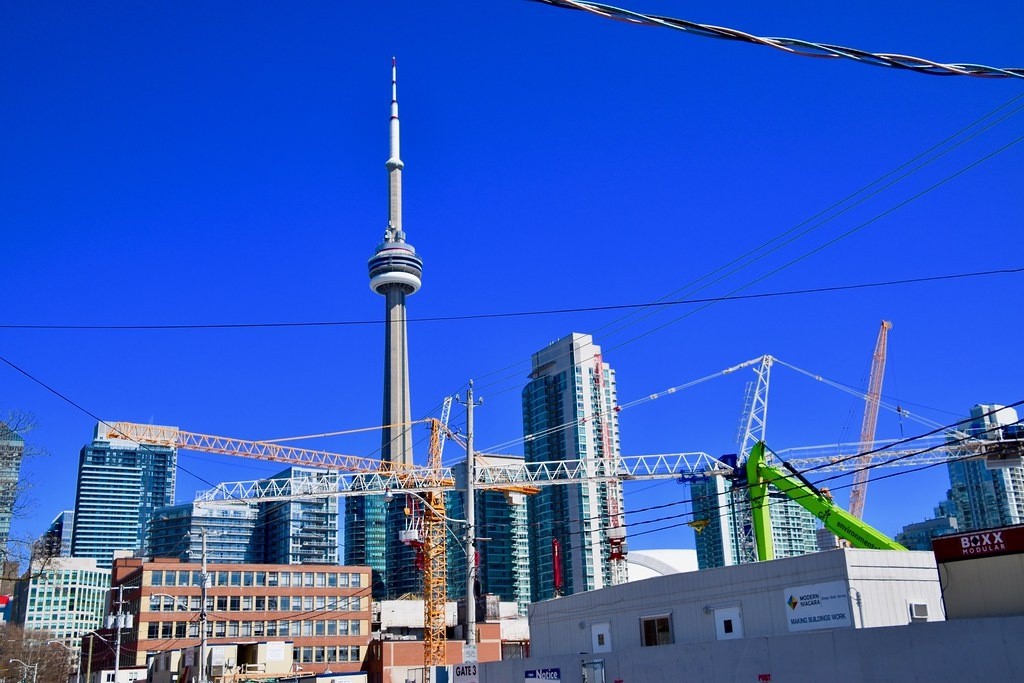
[194,395,734,617]
[727,320,1024,564]
[90,416,542,683]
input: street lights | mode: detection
[10,659,38,683]
[149,592,202,683]
[47,640,82,683]
[79,628,139,683]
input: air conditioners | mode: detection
[910,603,929,618]
